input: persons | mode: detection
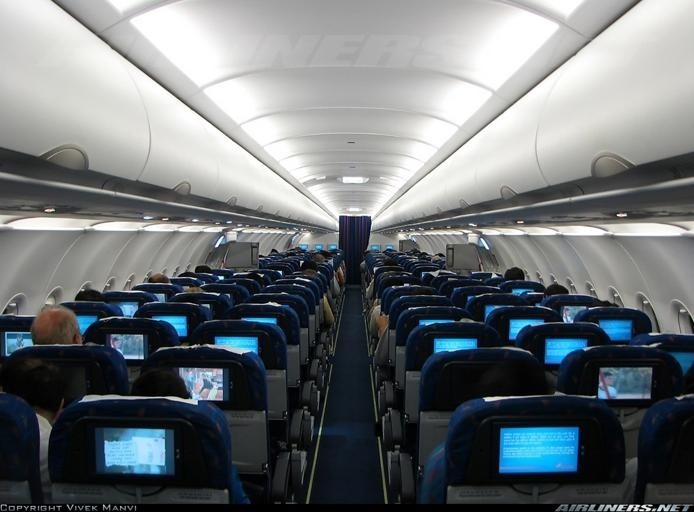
[300,249,347,325]
[371,316,390,353]
[368,298,381,338]
[542,283,569,301]
[412,247,445,264]
[130,370,250,504]
[31,305,84,346]
[74,263,213,300]
[504,268,525,282]
[1,351,69,503]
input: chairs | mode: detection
[0,247,346,506]
[360,247,693,503]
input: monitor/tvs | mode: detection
[240,314,277,325]
[216,335,260,358]
[298,244,338,250]
[148,315,188,338]
[153,293,166,304]
[94,425,178,479]
[111,301,137,316]
[371,245,393,252]
[172,366,229,402]
[77,314,99,338]
[105,332,145,361]
[4,331,37,356]
[404,286,694,507]
